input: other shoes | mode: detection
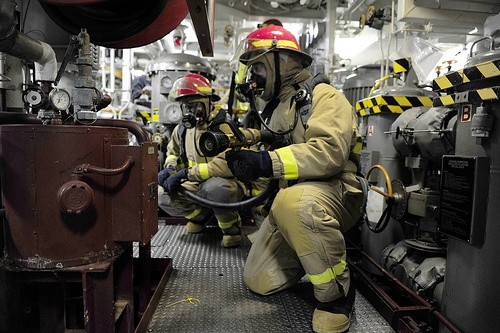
[186,210,214,233]
[221,220,241,247]
[312,293,355,333]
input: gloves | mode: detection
[163,168,185,195]
[158,167,173,187]
[225,150,273,180]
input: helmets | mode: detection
[239,25,312,69]
[174,74,221,102]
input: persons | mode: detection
[156,73,270,248]
[226,26,364,333]
